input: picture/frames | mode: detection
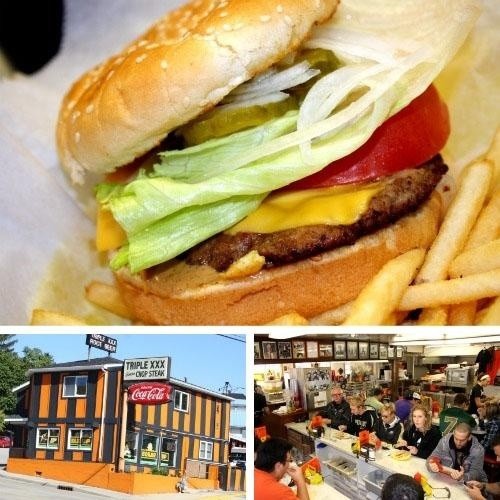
[254,340,405,360]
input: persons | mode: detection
[255,368,500,499]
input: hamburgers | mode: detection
[56,1,490,325]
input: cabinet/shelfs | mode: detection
[272,405,306,446]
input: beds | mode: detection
[285,410,472,500]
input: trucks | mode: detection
[228,447,247,470]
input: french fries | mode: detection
[298,137,499,330]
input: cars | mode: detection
[0,435,14,447]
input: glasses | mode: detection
[432,487,450,498]
